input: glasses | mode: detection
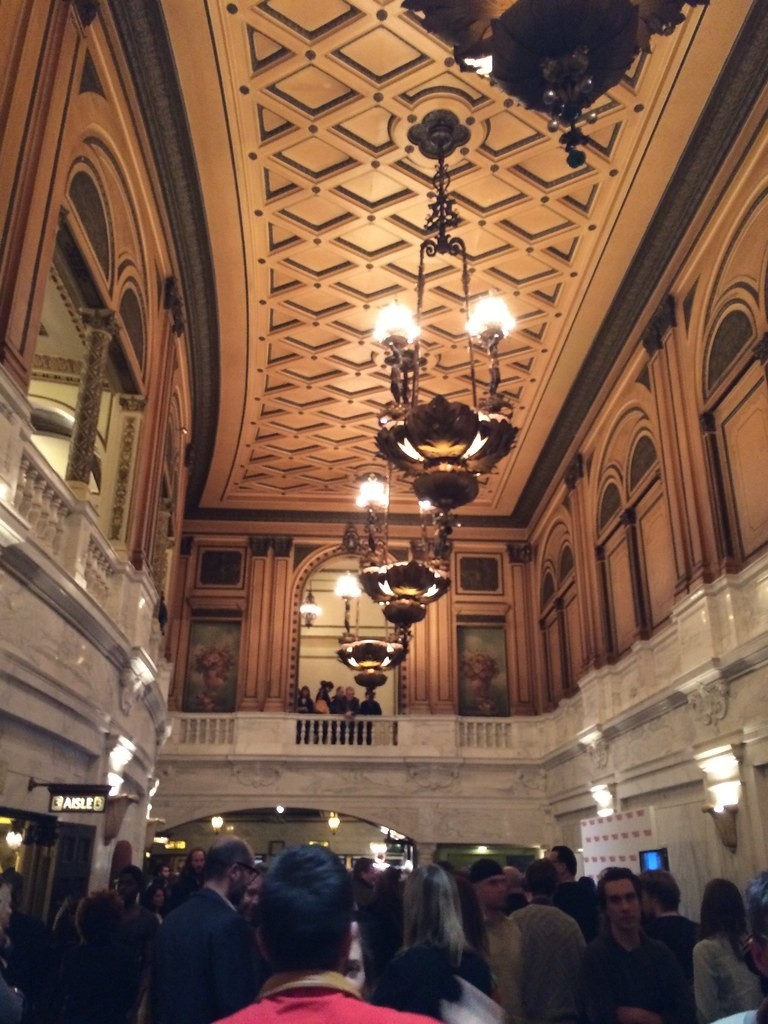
[236,861,260,882]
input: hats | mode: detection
[468,859,502,884]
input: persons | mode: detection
[692,877,763,1024]
[208,844,444,1024]
[507,860,584,1024]
[548,845,601,925]
[638,869,701,980]
[0,838,531,1024]
[368,863,493,1024]
[709,871,768,1024]
[576,865,689,1024]
[296,680,382,746]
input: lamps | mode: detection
[145,818,166,851]
[211,815,224,835]
[373,110,523,534]
[702,804,738,854]
[333,570,415,669]
[6,819,26,850]
[106,793,140,845]
[341,460,460,653]
[328,811,341,836]
[299,578,322,627]
[400,0,712,170]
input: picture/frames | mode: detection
[455,551,506,596]
[455,624,511,718]
[181,619,242,713]
[195,545,247,590]
